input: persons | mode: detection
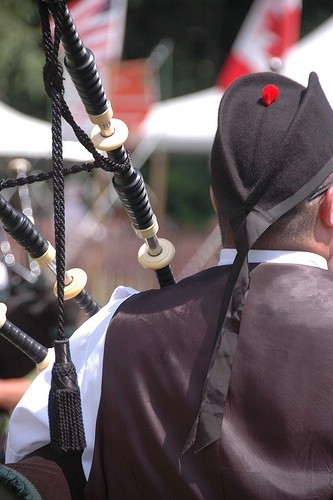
[3,70,333,500]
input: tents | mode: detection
[139,15,331,228]
[0,103,108,167]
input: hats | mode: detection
[211,71,333,254]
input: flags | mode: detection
[216,2,301,90]
[49,0,125,122]
